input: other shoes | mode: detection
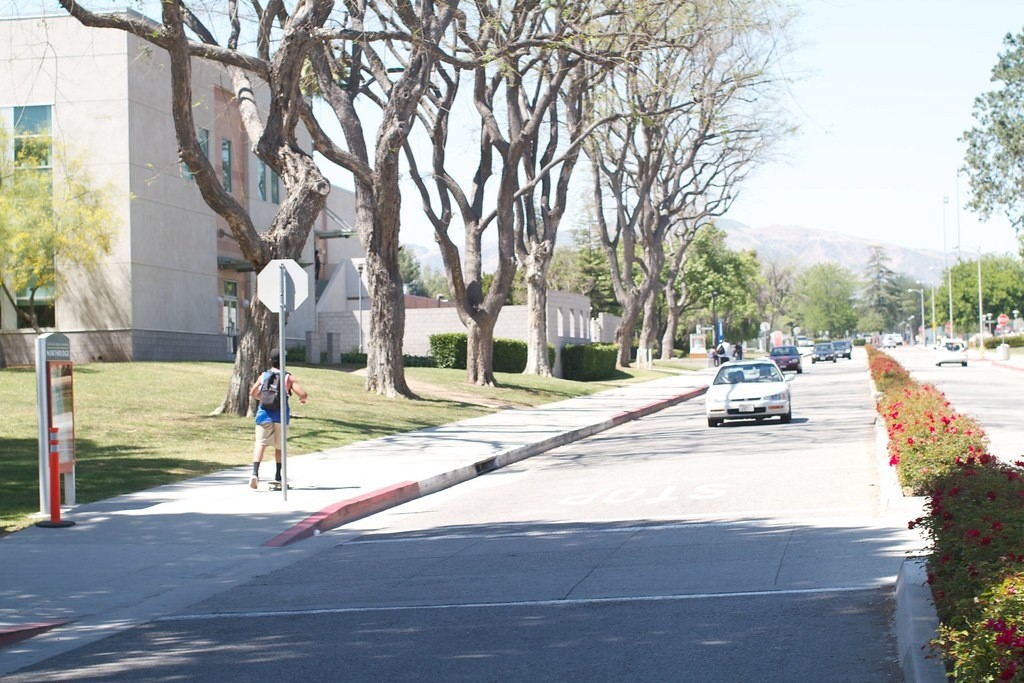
[274,477,288,483]
[250,475,258,489]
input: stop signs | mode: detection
[997,314,1009,326]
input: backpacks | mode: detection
[260,370,291,410]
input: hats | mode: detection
[270,349,287,362]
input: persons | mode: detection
[731,371,744,382]
[720,339,730,364]
[315,250,325,281]
[708,346,719,367]
[757,368,772,378]
[733,342,742,360]
[250,348,307,489]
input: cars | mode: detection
[705,357,796,427]
[809,343,837,364]
[768,346,803,374]
[858,333,904,349]
[829,340,851,359]
[934,338,969,368]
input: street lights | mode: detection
[928,265,953,342]
[915,279,937,348]
[357,264,364,352]
[907,287,926,345]
[952,243,983,349]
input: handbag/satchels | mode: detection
[717,344,724,354]
